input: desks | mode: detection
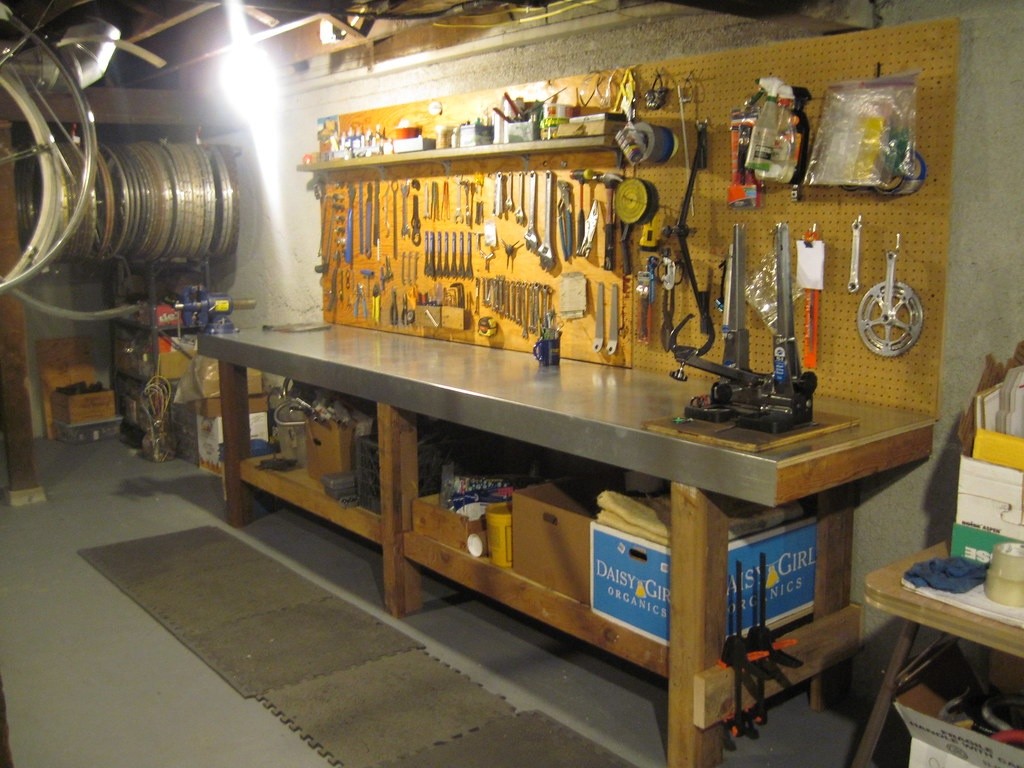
[850,541,1024,768]
[198,325,934,768]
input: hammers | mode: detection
[570,169,594,256]
[592,172,625,271]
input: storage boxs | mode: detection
[136,304,182,328]
[171,400,202,465]
[117,334,198,379]
[590,515,814,648]
[513,477,616,606]
[54,416,123,443]
[894,664,1024,768]
[302,413,355,480]
[393,137,436,154]
[356,436,457,515]
[411,492,487,553]
[949,394,1023,566]
[503,122,540,143]
[57,387,115,424]
[460,124,493,147]
[569,112,634,136]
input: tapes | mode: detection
[984,542,1024,608]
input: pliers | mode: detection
[354,283,367,318]
[389,285,398,326]
[401,293,408,325]
